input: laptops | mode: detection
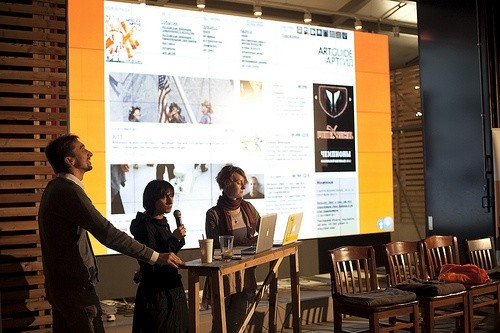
[234,213,304,255]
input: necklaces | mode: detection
[235,212,241,223]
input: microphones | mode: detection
[173,210,186,245]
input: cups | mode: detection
[199,239,213,264]
[218,236,234,262]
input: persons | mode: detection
[129,178,190,333]
[129,102,214,124]
[38,134,186,333]
[242,176,264,199]
[202,166,262,333]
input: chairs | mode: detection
[383,241,469,333]
[423,235,500,333]
[330,246,420,333]
[465,237,500,279]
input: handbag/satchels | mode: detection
[438,264,491,284]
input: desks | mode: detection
[183,240,303,333]
